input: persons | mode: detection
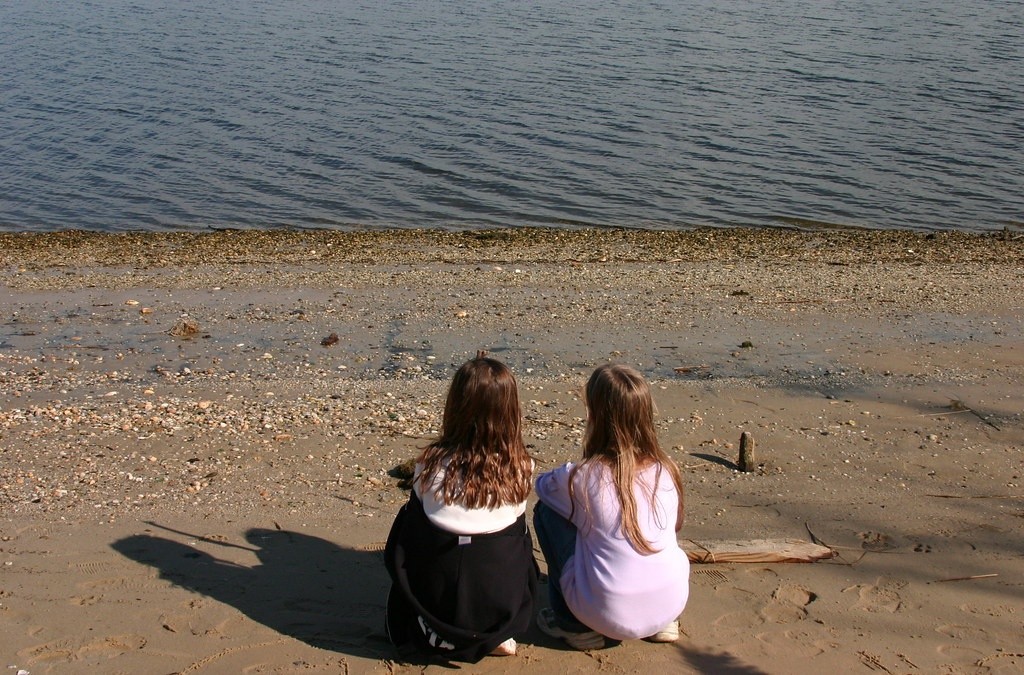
[532,362,690,650]
[384,358,540,664]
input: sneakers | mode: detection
[537,607,605,650]
[649,621,679,642]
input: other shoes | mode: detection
[487,637,516,655]
